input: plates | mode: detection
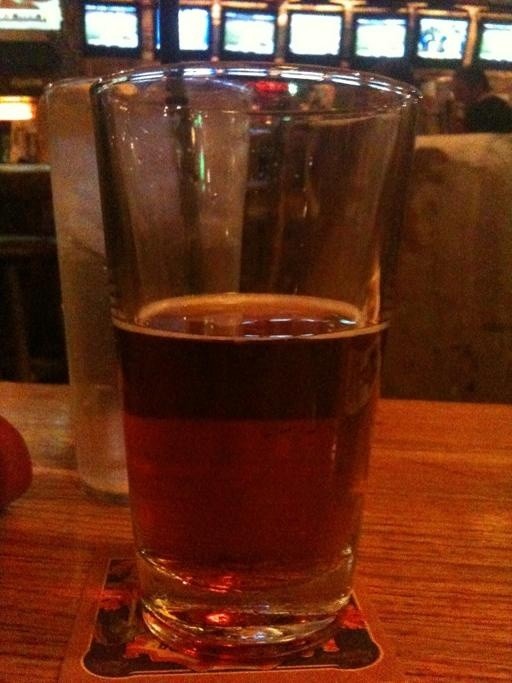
[2,414,32,510]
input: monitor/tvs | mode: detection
[411,14,471,68]
[349,12,410,72]
[218,5,278,62]
[471,18,512,71]
[284,9,345,67]
[81,1,143,60]
[153,2,212,60]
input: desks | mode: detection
[0,376,512,679]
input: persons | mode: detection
[448,64,512,132]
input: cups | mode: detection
[91,68,423,655]
[46,81,132,497]
